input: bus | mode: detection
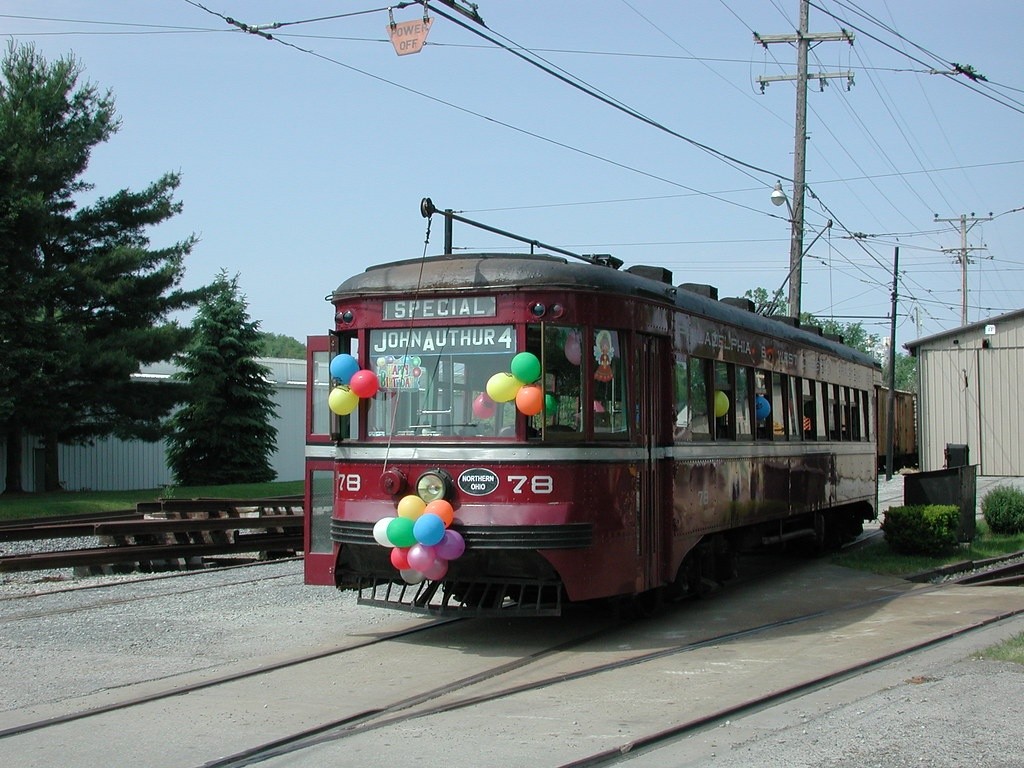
[303,252,883,620]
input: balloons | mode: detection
[329,386,359,415]
[564,333,582,365]
[373,494,464,585]
[350,370,379,397]
[511,352,540,385]
[540,394,557,417]
[473,395,496,418]
[486,373,525,404]
[516,385,542,416]
[755,396,771,419]
[715,391,729,417]
[329,353,359,384]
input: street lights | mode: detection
[772,179,805,320]
